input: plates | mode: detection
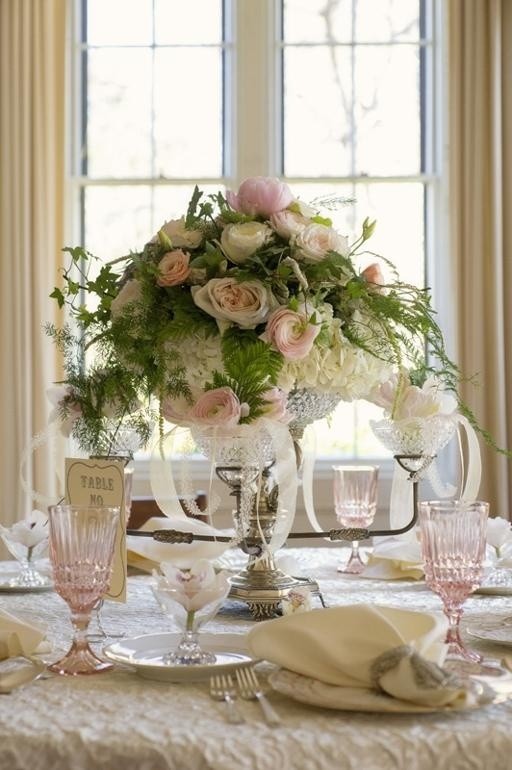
[102,630,267,685]
[464,609,512,647]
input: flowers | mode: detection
[37,175,482,457]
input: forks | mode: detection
[232,665,283,733]
[208,673,247,727]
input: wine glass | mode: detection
[417,499,490,665]
[45,504,123,677]
[148,582,233,667]
[0,533,51,590]
[330,464,381,575]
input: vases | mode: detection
[159,390,341,576]
